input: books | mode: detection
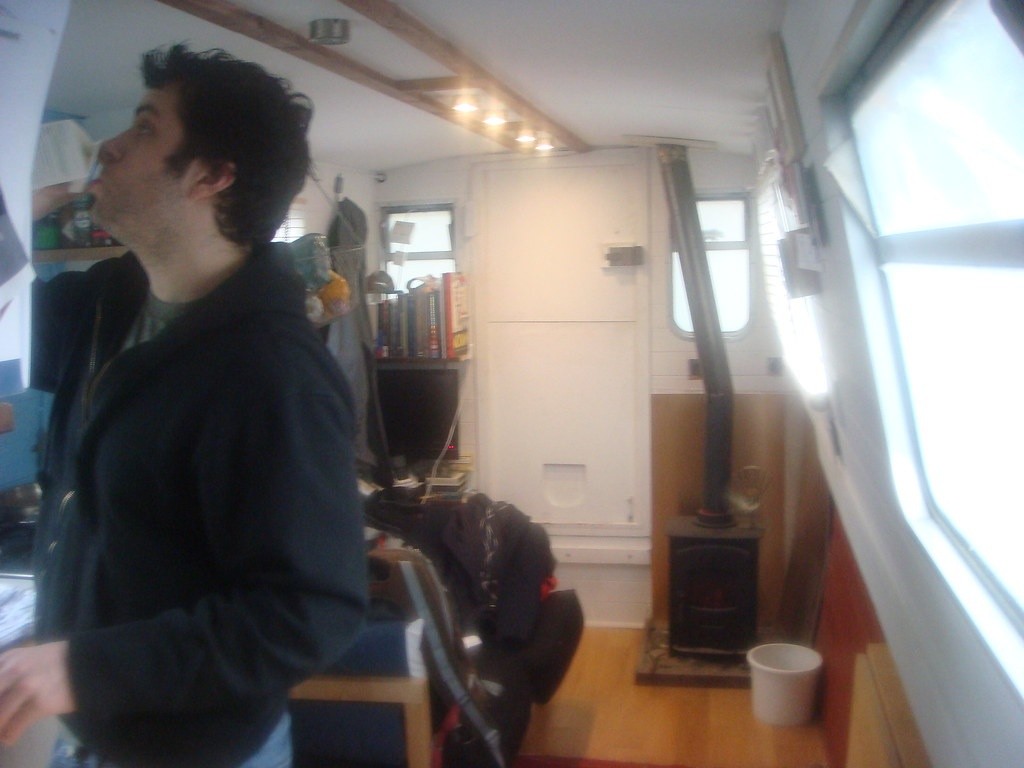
[397,458,474,493]
[368,272,469,362]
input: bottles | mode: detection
[70,196,92,247]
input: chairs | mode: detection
[289,619,433,768]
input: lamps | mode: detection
[454,93,555,151]
[311,19,351,48]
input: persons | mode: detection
[0,43,371,768]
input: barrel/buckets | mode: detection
[746,643,823,727]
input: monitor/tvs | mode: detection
[366,357,459,463]
